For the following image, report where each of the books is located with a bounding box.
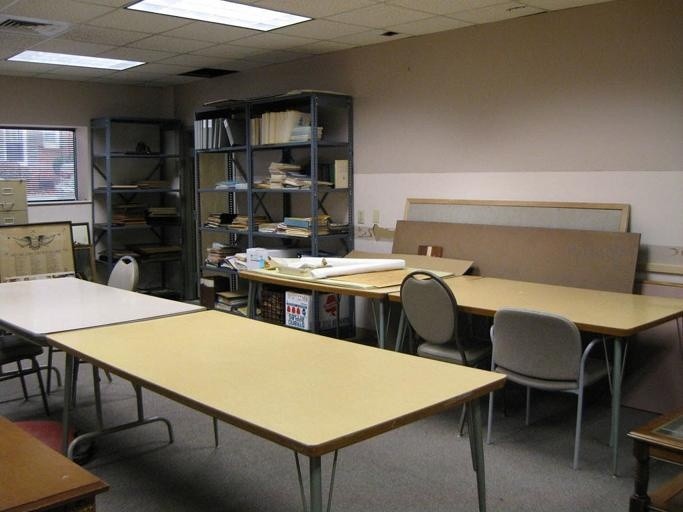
[191,111,346,319]
[97,179,185,302]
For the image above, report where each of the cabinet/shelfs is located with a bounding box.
[88,116,187,301]
[237,259,453,349]
[190,105,249,319]
[0,178,29,225]
[247,92,353,338]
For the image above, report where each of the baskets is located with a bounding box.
[261,288,284,323]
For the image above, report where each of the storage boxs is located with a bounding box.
[286,289,354,329]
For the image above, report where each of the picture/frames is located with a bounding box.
[402,196,633,235]
[0,221,78,279]
[74,245,97,284]
[71,221,91,247]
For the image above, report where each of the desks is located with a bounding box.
[389,271,681,478]
[624,403,682,511]
[47,308,507,507]
[0,412,113,510]
[0,274,207,449]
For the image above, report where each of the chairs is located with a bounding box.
[105,256,142,291]
[398,273,491,438]
[487,309,628,469]
[1,330,54,416]
